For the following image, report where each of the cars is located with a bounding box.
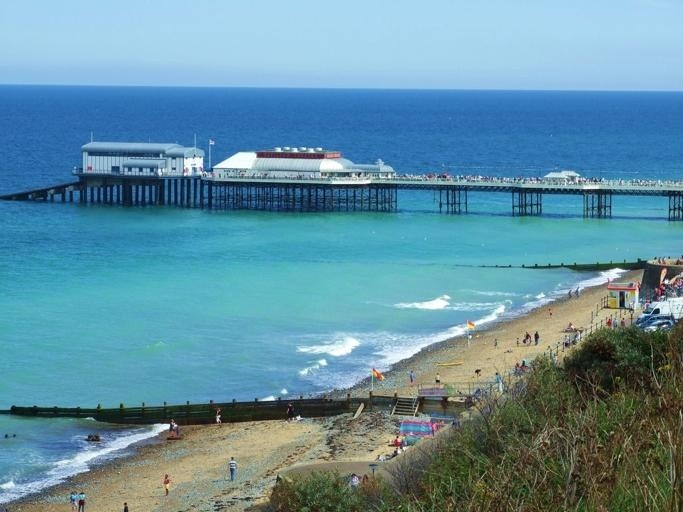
[635,286,682,334]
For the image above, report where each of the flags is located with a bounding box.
[372,367,384,381]
[467,320,475,330]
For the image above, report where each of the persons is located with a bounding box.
[376,435,406,461]
[216,408,222,424]
[169,419,181,438]
[496,372,503,394]
[123,503,128,512]
[70,491,86,512]
[431,422,437,437]
[409,370,414,386]
[472,369,481,378]
[435,373,441,384]
[494,331,539,376]
[163,473,170,496]
[228,457,239,481]
[549,256,683,349]
[286,402,294,422]
